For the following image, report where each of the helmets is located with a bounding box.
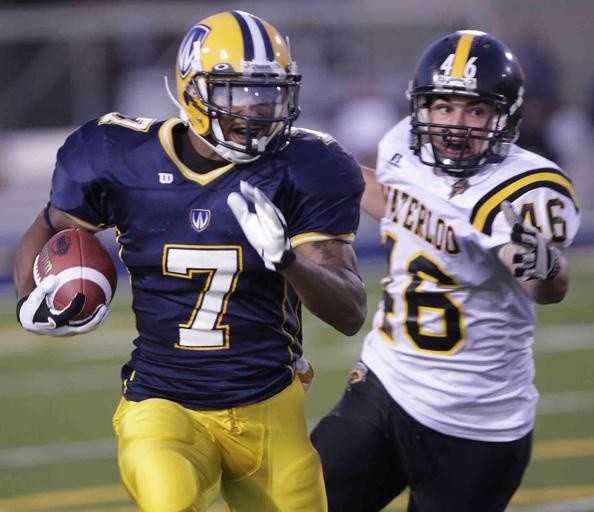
[407,28,527,172]
[176,10,301,163]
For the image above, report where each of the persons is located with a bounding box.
[11,9,367,512]
[307,30,583,512]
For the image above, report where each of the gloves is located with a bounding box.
[500,199,561,284]
[14,275,108,339]
[228,178,297,272]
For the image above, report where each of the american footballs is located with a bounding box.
[32,227,117,321]
